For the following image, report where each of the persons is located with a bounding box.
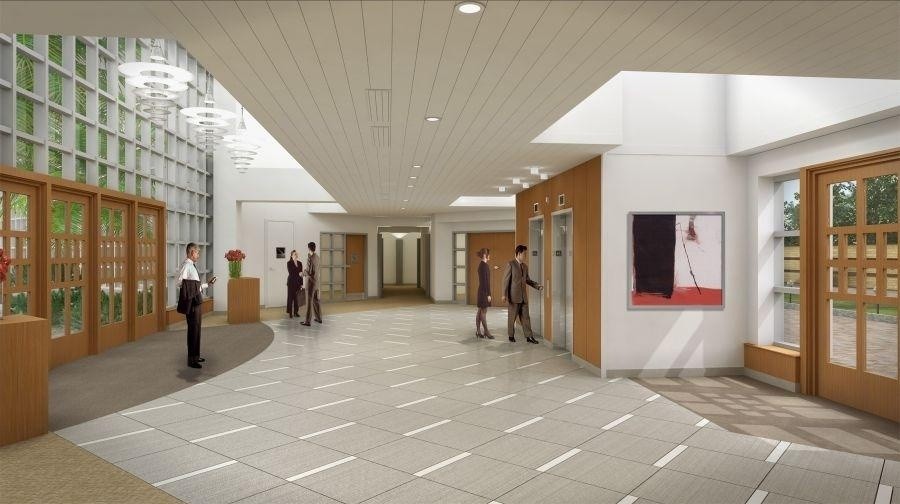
[178,242,209,370]
[287,250,303,319]
[501,245,543,344]
[475,248,494,339]
[300,242,323,327]
